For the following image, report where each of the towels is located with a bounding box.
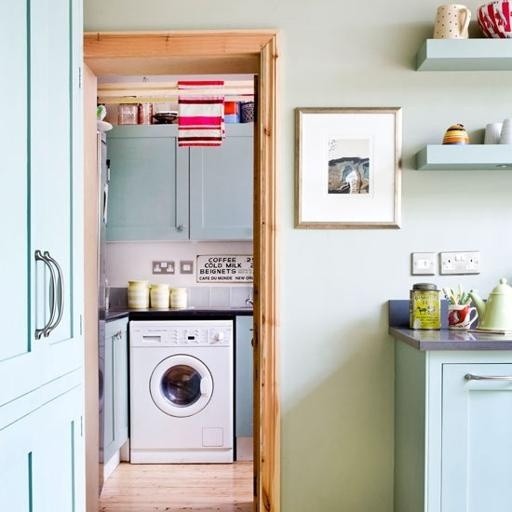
[177,80,225,147]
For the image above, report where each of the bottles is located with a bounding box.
[150,283,170,309]
[126,280,149,310]
[408,282,442,331]
[169,287,189,308]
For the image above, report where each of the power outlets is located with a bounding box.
[411,252,484,275]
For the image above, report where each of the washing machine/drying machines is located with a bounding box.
[129,319,235,465]
[98,320,106,463]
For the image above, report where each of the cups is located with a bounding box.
[482,123,501,144]
[446,304,481,332]
[498,117,512,144]
[431,3,472,40]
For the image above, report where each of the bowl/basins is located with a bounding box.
[441,122,469,145]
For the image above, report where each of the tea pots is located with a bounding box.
[466,276,511,334]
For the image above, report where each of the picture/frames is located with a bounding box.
[294,105,403,233]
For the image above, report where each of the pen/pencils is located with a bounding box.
[441,283,474,305]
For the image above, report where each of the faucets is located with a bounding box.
[245,300,253,304]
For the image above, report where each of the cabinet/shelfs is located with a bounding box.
[107,122,254,242]
[394,340,512,512]
[2,0,86,512]
